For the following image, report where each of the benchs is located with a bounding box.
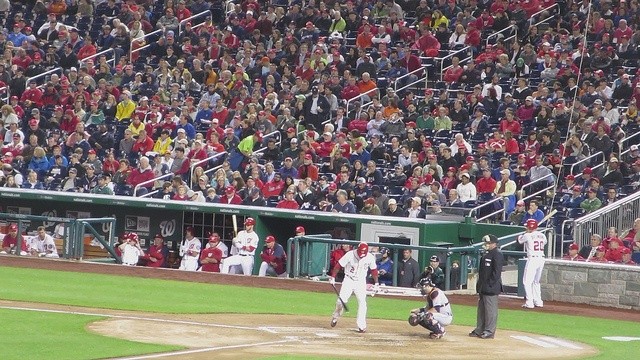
[0,236,117,264]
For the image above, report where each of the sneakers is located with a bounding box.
[522,304,534,309]
[481,333,494,339]
[430,331,446,339]
[536,305,543,308]
[469,331,479,337]
[359,327,366,333]
[331,318,337,327]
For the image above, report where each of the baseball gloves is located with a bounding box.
[408,312,427,326]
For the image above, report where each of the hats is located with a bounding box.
[542,42,550,46]
[411,197,421,204]
[525,96,533,101]
[620,248,630,254]
[307,124,316,131]
[90,102,97,106]
[264,236,274,242]
[305,154,312,159]
[462,173,470,178]
[287,127,296,134]
[11,96,18,100]
[406,121,416,127]
[211,38,218,44]
[13,134,20,138]
[249,158,258,163]
[212,119,218,124]
[4,151,13,158]
[330,183,337,189]
[177,139,188,145]
[481,168,491,174]
[129,233,138,241]
[482,234,499,242]
[408,129,415,134]
[573,186,580,191]
[447,167,456,172]
[389,199,396,205]
[596,246,605,251]
[580,169,591,174]
[68,27,81,32]
[324,132,332,137]
[122,234,128,239]
[68,168,77,173]
[296,226,304,232]
[424,90,432,94]
[505,93,513,98]
[29,120,38,127]
[594,99,603,106]
[568,244,577,249]
[630,145,638,151]
[564,175,574,179]
[420,278,436,286]
[355,142,361,149]
[225,187,234,192]
[428,155,437,160]
[358,243,368,257]
[89,150,96,154]
[11,225,17,231]
[594,69,604,76]
[524,219,537,229]
[247,3,256,11]
[149,113,157,117]
[258,111,266,116]
[466,156,475,162]
[154,233,162,238]
[425,142,431,147]
[244,218,255,225]
[357,178,365,184]
[590,178,599,182]
[210,236,217,241]
[607,237,619,241]
[58,32,64,36]
[364,198,374,203]
[597,116,604,122]
[587,189,597,193]
[429,256,439,261]
[610,157,617,162]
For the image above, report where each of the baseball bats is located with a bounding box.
[331,284,349,312]
[537,209,558,226]
[232,214,237,238]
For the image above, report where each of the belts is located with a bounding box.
[239,254,251,256]
[127,264,136,266]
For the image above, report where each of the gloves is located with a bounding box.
[330,278,335,286]
[417,312,428,322]
[411,309,420,314]
[370,283,379,296]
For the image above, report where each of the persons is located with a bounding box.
[0,0,640,226]
[31,226,59,258]
[469,234,503,339]
[330,239,353,281]
[373,247,393,286]
[450,260,461,291]
[223,218,260,276]
[142,234,169,268]
[409,278,453,339]
[115,233,145,266]
[0,224,26,256]
[563,218,640,267]
[258,236,287,277]
[518,219,547,308]
[397,248,421,288]
[419,255,445,290]
[197,232,228,273]
[113,231,130,256]
[329,241,379,333]
[178,227,201,271]
[291,227,305,272]
[198,237,222,273]
